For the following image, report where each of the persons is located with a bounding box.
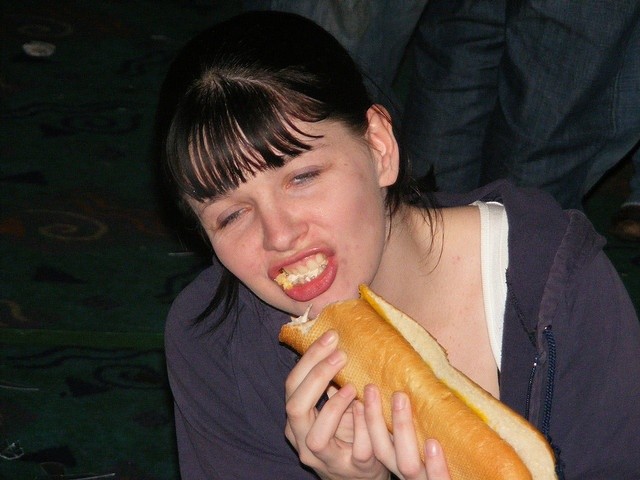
[164,11,640,479]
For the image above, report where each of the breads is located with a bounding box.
[278,282,559,480]
